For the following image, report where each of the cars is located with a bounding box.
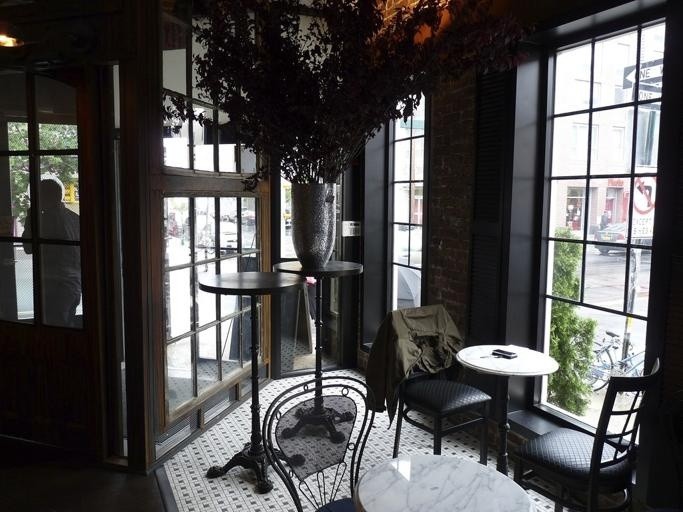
[595,224,627,253]
[242,212,255,226]
[168,213,237,255]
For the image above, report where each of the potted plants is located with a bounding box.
[167,0,455,269]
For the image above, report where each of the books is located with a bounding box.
[491,348,517,360]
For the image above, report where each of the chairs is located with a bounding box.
[522,358,661,512]
[367,303,493,464]
[262,376,377,512]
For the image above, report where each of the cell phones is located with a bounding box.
[492,348,517,359]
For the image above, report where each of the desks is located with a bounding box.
[354,454,530,512]
[199,260,363,494]
[455,344,560,475]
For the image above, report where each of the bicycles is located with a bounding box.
[574,330,645,391]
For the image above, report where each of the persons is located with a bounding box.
[599,208,609,231]
[570,200,581,222]
[20,175,82,329]
